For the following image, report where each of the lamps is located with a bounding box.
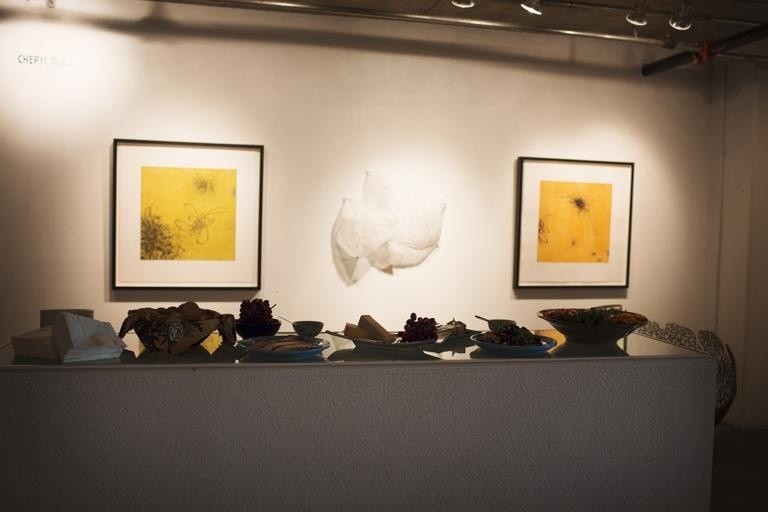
[624,1,693,31]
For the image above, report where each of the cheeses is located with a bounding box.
[437,321,467,341]
[345,315,396,344]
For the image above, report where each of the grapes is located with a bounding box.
[477,324,542,346]
[399,313,437,341]
[237,298,276,325]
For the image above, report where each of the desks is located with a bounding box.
[0,319,718,511]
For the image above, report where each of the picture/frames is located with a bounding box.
[516,157,634,288]
[112,138,261,287]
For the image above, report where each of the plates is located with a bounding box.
[330,313,437,354]
[473,315,555,354]
[239,333,329,360]
[437,320,484,345]
[537,307,648,346]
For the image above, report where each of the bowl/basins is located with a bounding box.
[234,319,281,339]
[291,318,323,338]
